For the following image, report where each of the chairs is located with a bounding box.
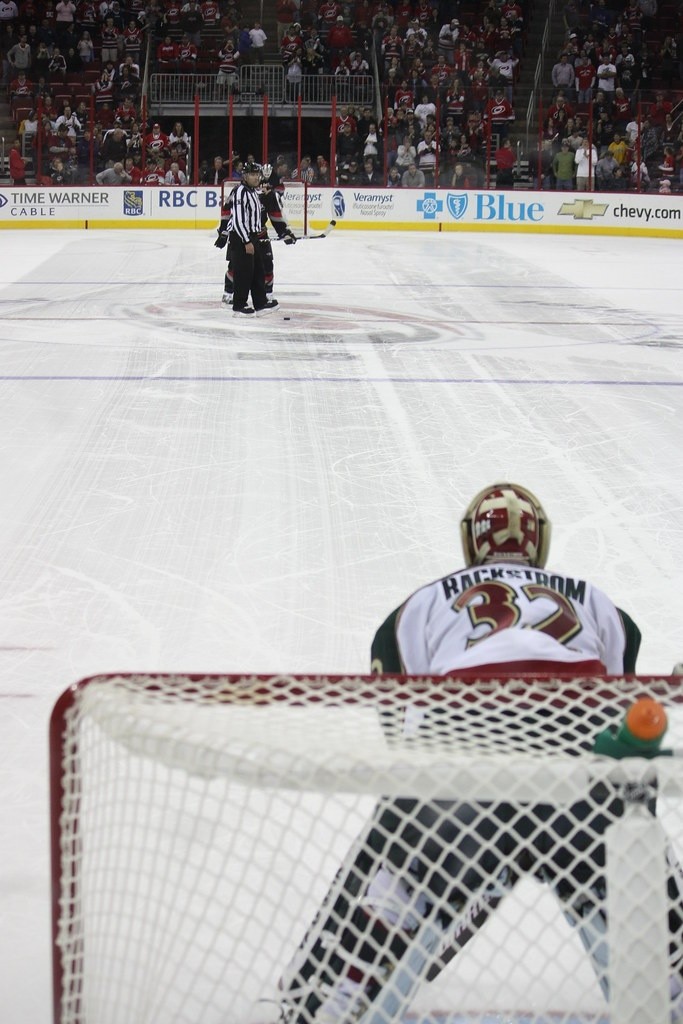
[0,0,683,191]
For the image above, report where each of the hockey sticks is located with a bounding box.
[259,219,336,243]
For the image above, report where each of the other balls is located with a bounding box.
[284,317,290,320]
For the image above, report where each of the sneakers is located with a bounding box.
[265,292,273,301]
[232,305,256,318]
[221,293,234,309]
[255,299,280,317]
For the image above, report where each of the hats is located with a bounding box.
[153,123,160,129]
[113,119,121,125]
[660,180,671,186]
[350,160,357,166]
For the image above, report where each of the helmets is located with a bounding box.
[459,483,552,569]
[240,162,262,174]
[261,164,272,178]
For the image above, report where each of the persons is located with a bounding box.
[278,483,683,1024]
[574,138,598,191]
[608,133,635,163]
[164,163,187,185]
[659,180,671,193]
[552,142,578,191]
[0,0,683,198]
[249,21,267,65]
[95,163,132,185]
[216,39,239,92]
[10,140,27,185]
[495,139,517,189]
[215,162,297,318]
[402,164,425,187]
[529,139,551,190]
[236,25,253,79]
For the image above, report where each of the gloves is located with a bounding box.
[214,231,228,248]
[277,191,284,197]
[282,230,296,244]
